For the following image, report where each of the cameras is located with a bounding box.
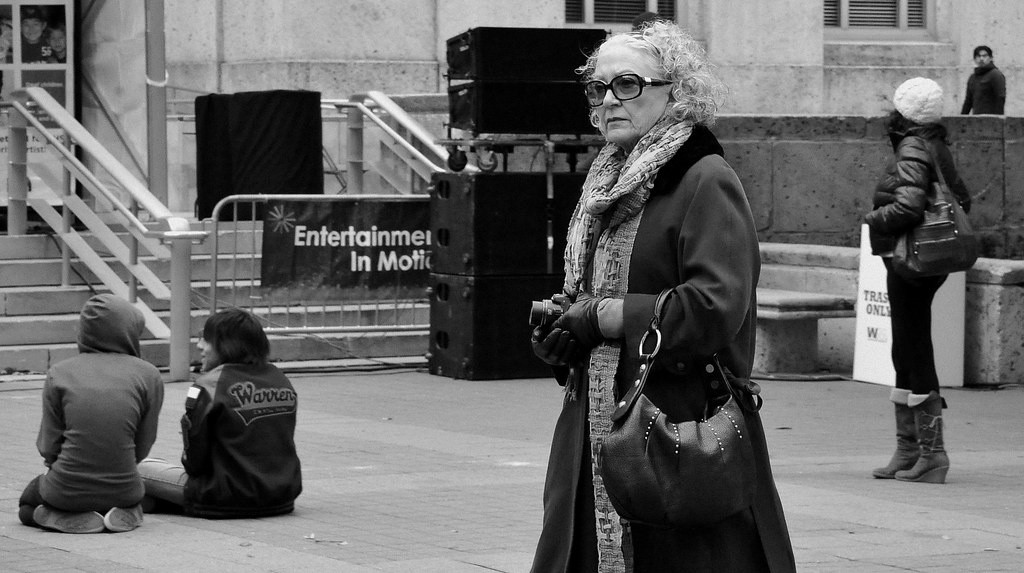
[530,296,573,339]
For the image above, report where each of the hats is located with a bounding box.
[892,76,946,126]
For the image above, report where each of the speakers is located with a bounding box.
[429,28,610,381]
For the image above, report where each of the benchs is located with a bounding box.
[756,288,856,374]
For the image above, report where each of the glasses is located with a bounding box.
[584,73,674,108]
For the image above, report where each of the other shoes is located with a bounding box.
[104,503,144,532]
[33,505,104,533]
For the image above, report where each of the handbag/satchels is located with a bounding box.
[597,286,763,530]
[892,135,979,281]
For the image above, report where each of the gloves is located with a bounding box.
[550,293,614,353]
[530,326,576,367]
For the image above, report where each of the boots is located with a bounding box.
[872,386,922,478]
[894,390,951,484]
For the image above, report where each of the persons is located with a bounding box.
[529,33,799,573]
[865,79,971,483]
[961,44,1007,116]
[135,308,303,520]
[18,294,164,532]
[20,6,68,65]
